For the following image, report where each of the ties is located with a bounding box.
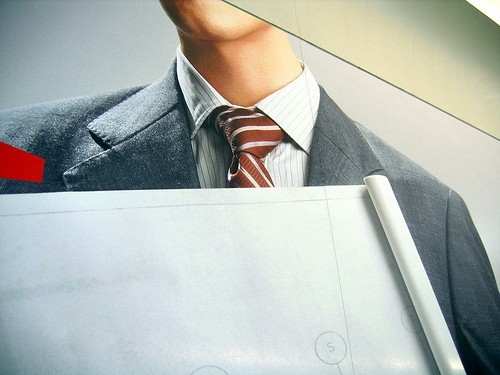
[201,104,288,187]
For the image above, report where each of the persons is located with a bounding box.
[0,0,500,374]
